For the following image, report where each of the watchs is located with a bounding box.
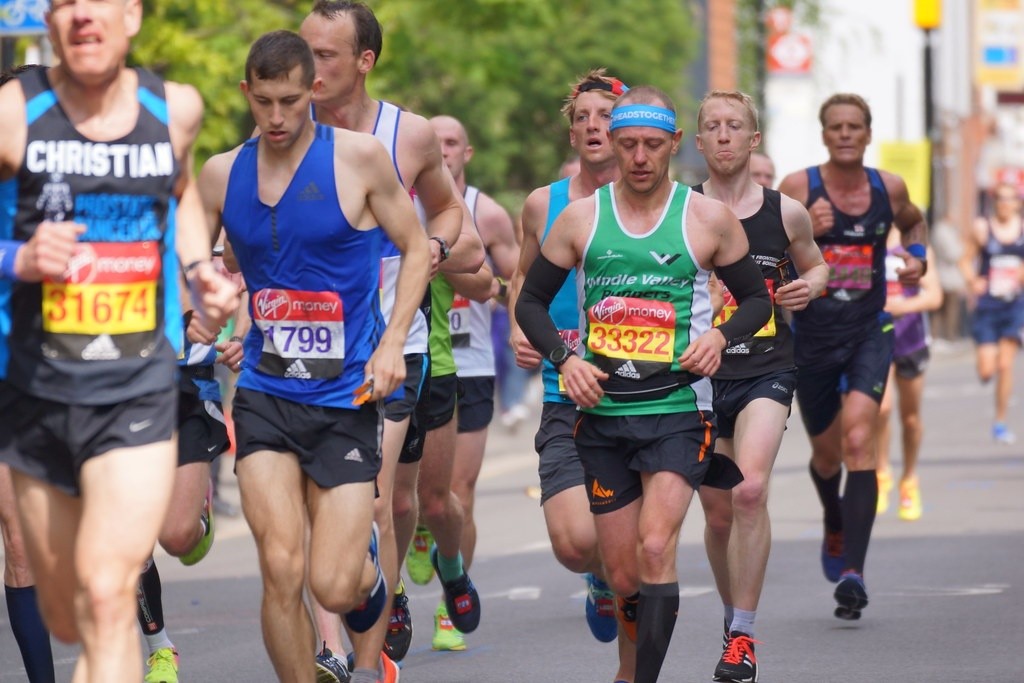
[549,344,578,374]
[429,237,450,263]
[494,277,507,302]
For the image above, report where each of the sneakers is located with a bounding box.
[383,576,413,662]
[430,543,480,634]
[179,477,216,566]
[432,601,466,651]
[833,570,869,620]
[712,616,759,683]
[820,497,847,582]
[145,648,180,683]
[343,520,388,633]
[586,572,617,642]
[406,524,436,585]
[876,470,893,515]
[897,475,921,521]
[616,591,641,643]
[315,640,403,683]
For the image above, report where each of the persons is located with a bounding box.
[509,67,942,683]
[0,0,520,683]
[958,183,1024,444]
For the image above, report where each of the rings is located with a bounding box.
[237,361,241,366]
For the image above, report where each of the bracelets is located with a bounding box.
[183,259,204,288]
[905,244,926,262]
[229,335,242,343]
[916,257,926,276]
[0,240,27,284]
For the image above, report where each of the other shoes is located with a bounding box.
[993,422,1012,445]
